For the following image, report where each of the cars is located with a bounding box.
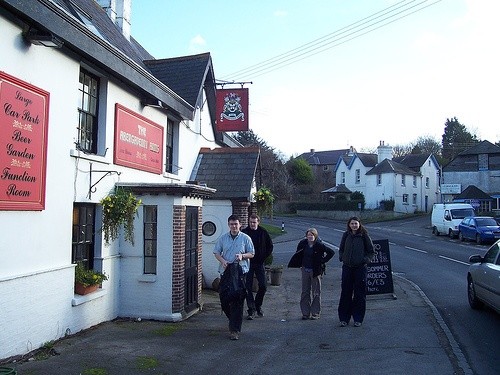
[459,216,500,245]
[466,239,500,314]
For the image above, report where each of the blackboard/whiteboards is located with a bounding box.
[366,239,394,296]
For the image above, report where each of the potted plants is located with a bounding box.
[269,264,284,286]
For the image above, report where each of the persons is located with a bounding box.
[241,214,273,320]
[214,215,255,339]
[338,217,374,328]
[288,228,335,320]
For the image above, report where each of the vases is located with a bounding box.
[75,282,99,296]
[259,200,271,207]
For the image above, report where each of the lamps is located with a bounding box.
[24,29,64,49]
[143,99,164,110]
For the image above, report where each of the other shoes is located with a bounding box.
[354,322,362,327]
[340,320,348,327]
[257,308,264,317]
[302,315,309,320]
[248,313,256,319]
[230,331,240,340]
[310,313,320,320]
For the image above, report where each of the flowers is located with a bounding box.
[74,264,110,287]
[95,192,141,247]
[253,186,278,204]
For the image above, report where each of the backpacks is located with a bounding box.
[219,259,248,301]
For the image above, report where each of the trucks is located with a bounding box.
[431,203,476,239]
